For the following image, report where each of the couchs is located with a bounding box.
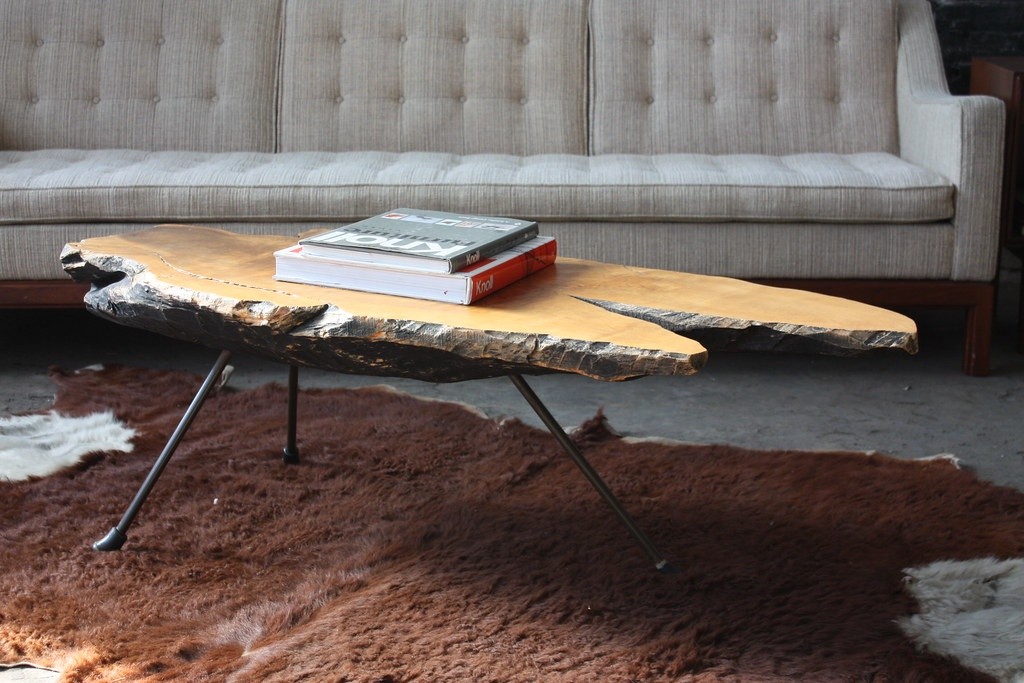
[0,0,1010,377]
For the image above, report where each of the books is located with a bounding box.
[275,205,559,306]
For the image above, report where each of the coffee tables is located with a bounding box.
[60,224,918,572]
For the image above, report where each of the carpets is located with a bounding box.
[0,359,1024,680]
